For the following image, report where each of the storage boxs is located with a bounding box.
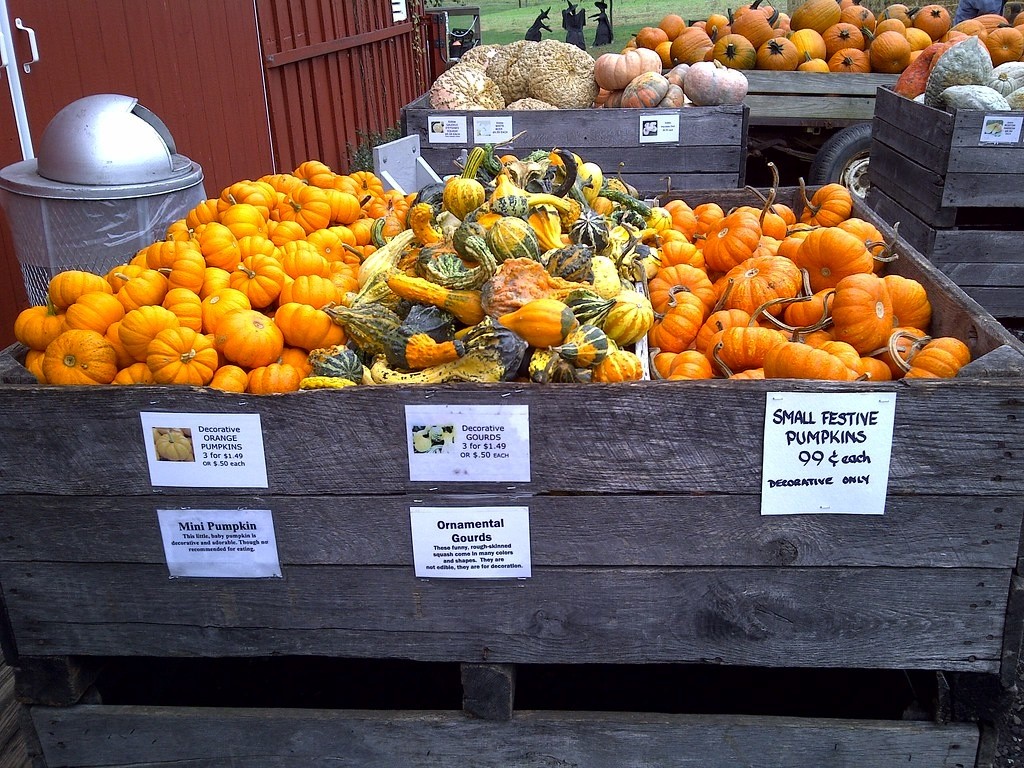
[2,81,1024,768]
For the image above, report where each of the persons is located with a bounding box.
[561,0,586,50]
[525,6,552,43]
[587,0,613,46]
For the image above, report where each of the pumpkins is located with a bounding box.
[429,0,1024,113]
[14,138,972,393]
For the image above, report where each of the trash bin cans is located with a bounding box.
[0,94,206,311]
